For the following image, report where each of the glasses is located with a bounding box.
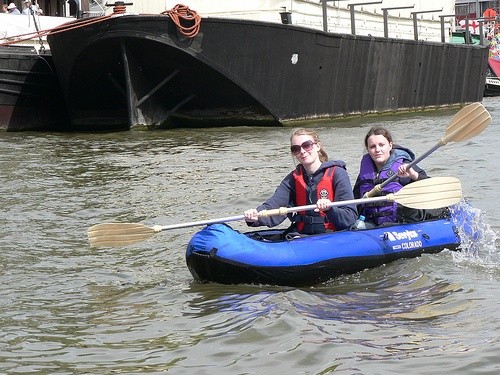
[291,140,317,155]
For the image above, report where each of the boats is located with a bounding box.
[46,0,491,129]
[185,202,482,290]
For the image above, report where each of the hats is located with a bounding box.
[24,0,31,3]
[8,3,17,9]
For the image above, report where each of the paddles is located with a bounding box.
[86,176,462,249]
[362,102,492,199]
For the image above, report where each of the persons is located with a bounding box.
[353,127,427,226]
[243,129,358,241]
[2,0,31,15]
